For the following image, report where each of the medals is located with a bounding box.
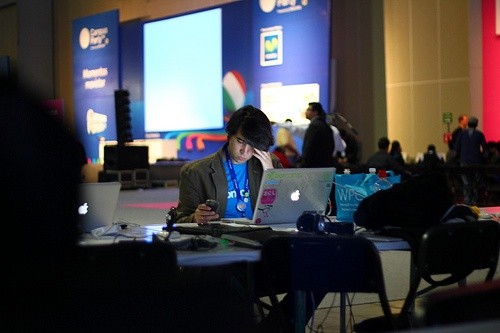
[236,201,247,212]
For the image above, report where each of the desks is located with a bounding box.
[80,216,410,333]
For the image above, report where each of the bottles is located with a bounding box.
[369,168,376,174]
[343,169,351,175]
[378,170,387,181]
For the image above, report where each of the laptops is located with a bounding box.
[221,166,337,225]
[78,181,127,234]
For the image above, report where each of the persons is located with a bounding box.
[175,105,292,224]
[366,137,412,174]
[415,115,500,205]
[301,101,362,173]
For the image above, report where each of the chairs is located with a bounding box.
[401,220,500,316]
[262,236,391,333]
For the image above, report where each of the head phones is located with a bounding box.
[296,213,354,235]
